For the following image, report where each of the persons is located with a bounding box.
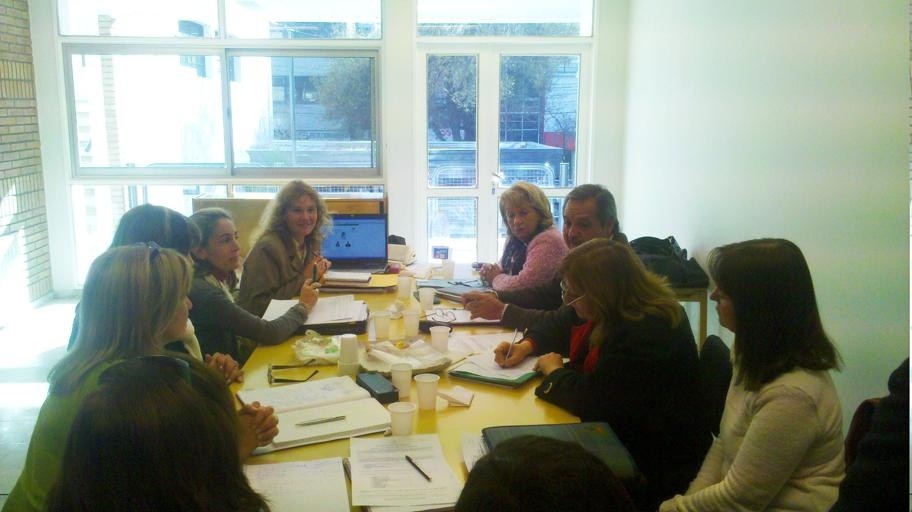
[659,238,850,510]
[43,241,280,512]
[462,183,631,328]
[185,210,321,365]
[453,432,638,512]
[497,235,708,497]
[46,355,268,511]
[237,178,331,331]
[469,182,568,293]
[107,205,245,391]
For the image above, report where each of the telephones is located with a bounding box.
[371,263,402,275]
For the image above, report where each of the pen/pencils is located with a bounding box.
[503,328,518,369]
[312,263,317,282]
[452,307,465,310]
[295,415,345,426]
[405,455,432,480]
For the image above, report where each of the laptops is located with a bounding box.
[318,213,388,274]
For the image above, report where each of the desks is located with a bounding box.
[672,286,708,358]
[228,276,582,512]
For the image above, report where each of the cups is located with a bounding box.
[429,326,451,350]
[374,312,390,339]
[403,309,419,337]
[398,276,411,296]
[390,363,412,398]
[387,402,417,436]
[443,261,454,280]
[338,334,360,373]
[420,288,434,308]
[414,373,440,412]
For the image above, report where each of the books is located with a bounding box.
[449,352,574,391]
[238,374,392,454]
[437,283,470,302]
[345,432,460,510]
[245,458,351,512]
[320,270,398,294]
[426,303,503,327]
[483,420,640,482]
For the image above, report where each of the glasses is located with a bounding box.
[421,308,457,323]
[134,240,163,262]
[561,280,588,307]
[266,359,320,385]
